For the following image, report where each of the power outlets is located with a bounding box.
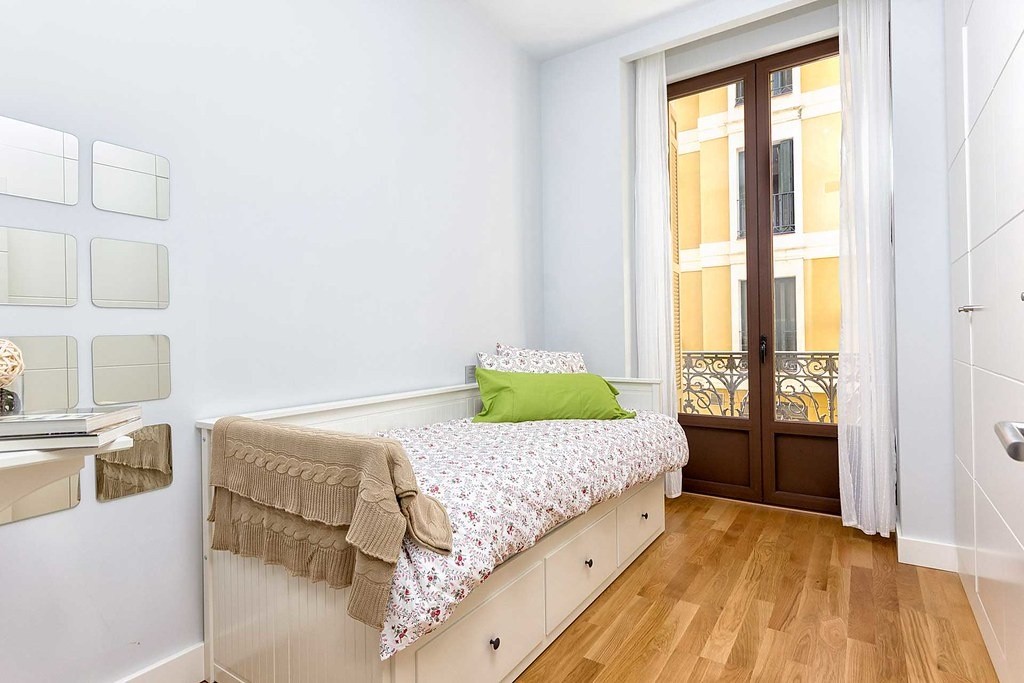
[466,365,476,383]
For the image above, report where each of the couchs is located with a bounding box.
[196,376,689,683]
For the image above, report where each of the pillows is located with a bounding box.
[472,343,636,422]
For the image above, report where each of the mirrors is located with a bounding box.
[91,334,170,405]
[0,336,79,413]
[0,115,79,205]
[0,472,82,526]
[90,237,170,308]
[91,139,170,220]
[1,226,78,307]
[96,424,174,502]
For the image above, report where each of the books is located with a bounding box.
[0,405,140,438]
[0,418,143,453]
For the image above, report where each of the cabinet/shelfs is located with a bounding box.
[946,0,1024,683]
